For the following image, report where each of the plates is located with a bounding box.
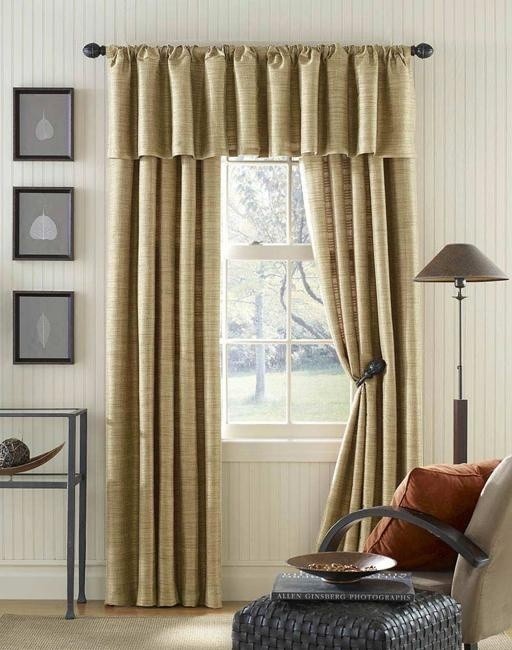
[286,551,398,583]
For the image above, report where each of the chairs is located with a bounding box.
[316,454,512,650]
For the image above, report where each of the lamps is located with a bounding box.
[412,243,509,466]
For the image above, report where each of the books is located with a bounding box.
[269,569,416,604]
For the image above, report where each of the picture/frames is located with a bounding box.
[12,87,74,364]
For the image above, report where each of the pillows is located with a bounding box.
[359,457,504,572]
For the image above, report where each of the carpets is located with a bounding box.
[0,609,241,649]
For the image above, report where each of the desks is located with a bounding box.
[231,582,463,649]
